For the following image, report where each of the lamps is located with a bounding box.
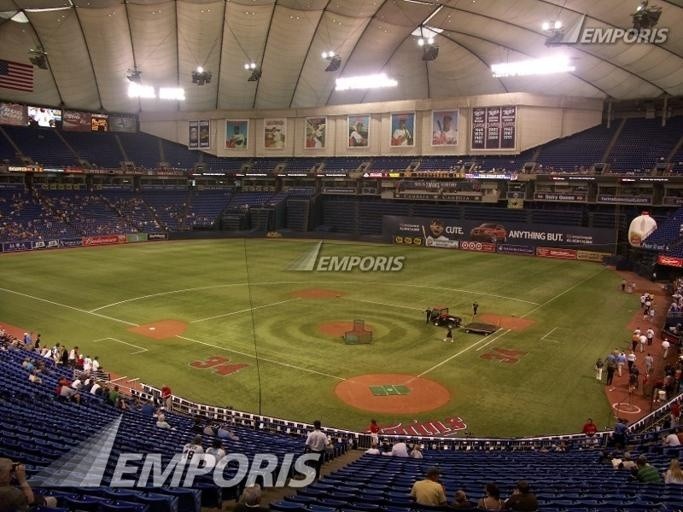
[543,0,662,49]
[127,66,263,86]
[28,49,49,71]
[421,47,439,61]
[324,55,341,72]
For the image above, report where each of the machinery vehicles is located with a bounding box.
[430,305,463,329]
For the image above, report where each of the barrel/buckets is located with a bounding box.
[627,211,657,248]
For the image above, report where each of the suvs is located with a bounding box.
[470,222,507,243]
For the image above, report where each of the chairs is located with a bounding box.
[0,114,683,258]
[0,345,683,512]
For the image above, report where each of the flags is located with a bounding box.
[0,59,33,93]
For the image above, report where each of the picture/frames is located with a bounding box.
[389,108,460,148]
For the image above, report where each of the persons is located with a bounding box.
[423,305,432,327]
[433,115,456,144]
[231,484,269,512]
[0,327,143,413]
[410,467,448,507]
[504,480,537,510]
[613,417,626,433]
[370,419,379,448]
[450,490,469,508]
[142,398,154,415]
[229,125,245,148]
[391,118,411,144]
[582,418,597,449]
[595,276,681,402]
[336,432,423,459]
[442,322,453,343]
[308,121,323,147]
[0,184,212,241]
[421,219,448,243]
[159,382,172,411]
[0,457,57,512]
[181,418,240,471]
[152,408,161,418]
[350,122,366,146]
[670,401,680,429]
[33,107,54,127]
[475,484,503,510]
[156,415,170,429]
[269,126,281,144]
[596,443,682,485]
[304,420,335,478]
[660,429,680,448]
[470,300,477,319]
